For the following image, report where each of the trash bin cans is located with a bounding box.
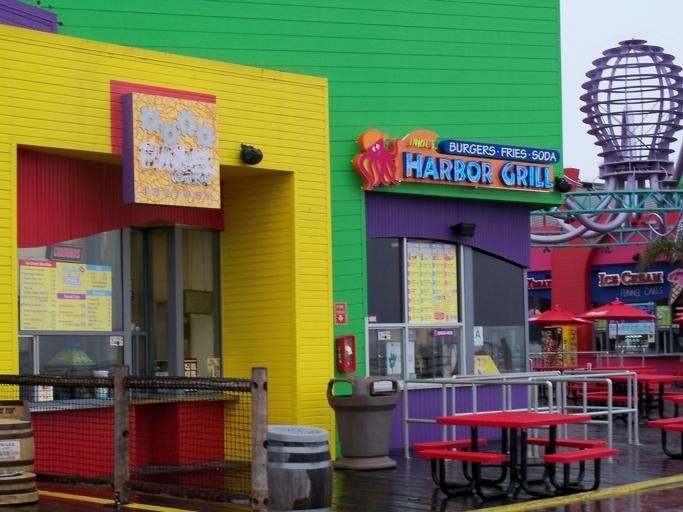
[327,375,400,470]
[263,424,333,512]
[0,400,39,512]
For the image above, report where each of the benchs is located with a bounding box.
[412,369,683,503]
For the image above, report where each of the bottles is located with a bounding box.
[545,328,559,366]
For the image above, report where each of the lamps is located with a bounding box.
[450,223,476,237]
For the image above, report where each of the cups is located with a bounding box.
[586,362,591,370]
[93,370,109,400]
